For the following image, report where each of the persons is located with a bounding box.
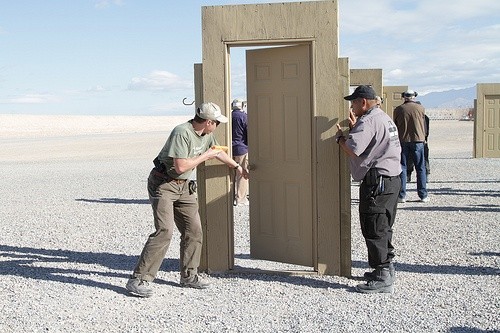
[229,99,249,205]
[125,103,249,296]
[391,89,431,203]
[337,85,404,293]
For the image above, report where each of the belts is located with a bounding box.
[155,170,184,184]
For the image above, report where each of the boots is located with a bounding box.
[355,263,396,294]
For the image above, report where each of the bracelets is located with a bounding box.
[233,164,240,170]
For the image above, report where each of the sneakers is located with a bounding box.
[178,274,210,289]
[126,275,154,297]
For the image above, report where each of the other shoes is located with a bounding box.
[398,196,406,204]
[420,197,430,202]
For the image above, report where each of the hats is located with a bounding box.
[233,99,242,109]
[196,102,229,124]
[343,85,377,101]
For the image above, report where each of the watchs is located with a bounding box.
[336,136,342,144]
